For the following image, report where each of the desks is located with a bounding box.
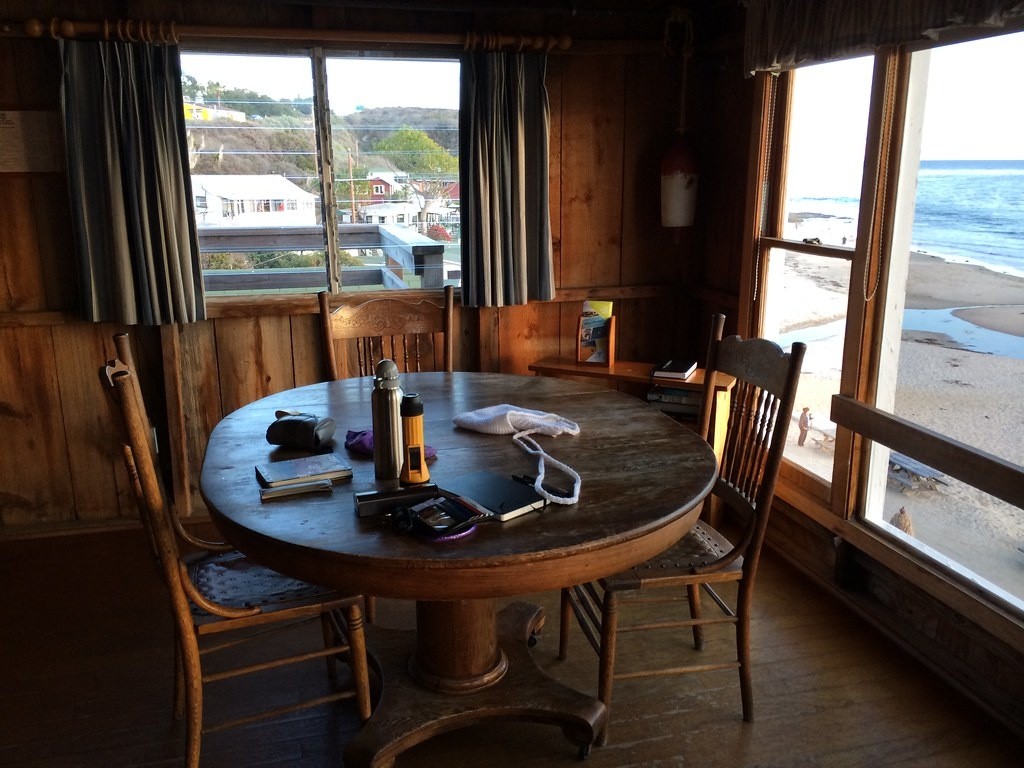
[818,428,836,450]
[889,452,945,495]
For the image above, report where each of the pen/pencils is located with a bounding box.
[511,473,535,487]
[522,473,571,496]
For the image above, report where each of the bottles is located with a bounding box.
[371,359,404,479]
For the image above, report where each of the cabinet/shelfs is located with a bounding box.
[527,355,738,534]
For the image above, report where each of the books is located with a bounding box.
[643,384,706,424]
[254,451,355,493]
[650,357,699,380]
[435,470,556,523]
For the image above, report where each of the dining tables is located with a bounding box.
[199,370,719,768]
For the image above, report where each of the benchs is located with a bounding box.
[811,436,836,452]
[888,466,950,490]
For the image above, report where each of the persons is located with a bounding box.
[797,407,813,446]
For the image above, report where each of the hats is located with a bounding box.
[455,404,579,507]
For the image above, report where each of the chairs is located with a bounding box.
[313,284,454,382]
[560,313,809,751]
[97,330,371,767]
